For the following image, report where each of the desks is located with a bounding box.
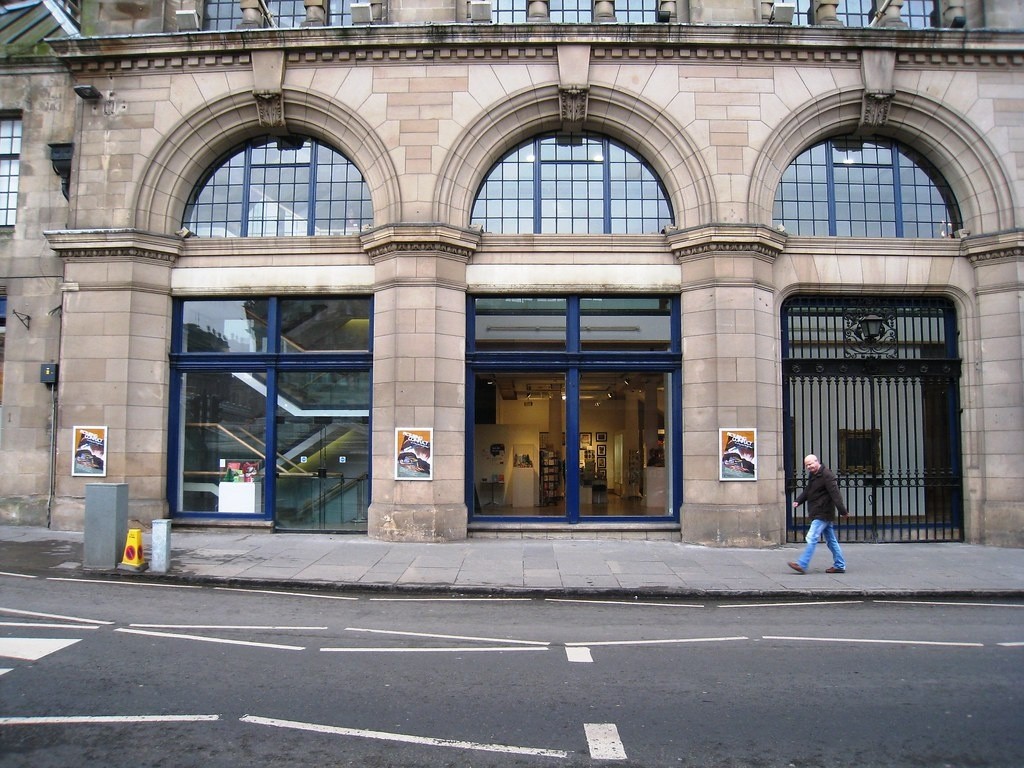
[512,467,534,507]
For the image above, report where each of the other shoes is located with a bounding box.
[826,566,845,572]
[787,562,805,573]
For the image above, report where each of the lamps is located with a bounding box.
[624,378,630,385]
[656,11,671,23]
[769,3,796,25]
[73,85,104,100]
[950,16,967,29]
[349,3,375,27]
[607,392,615,397]
[471,1,492,24]
[176,10,201,33]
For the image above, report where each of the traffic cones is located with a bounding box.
[117,528,148,570]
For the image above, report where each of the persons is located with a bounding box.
[647,436,665,467]
[788,454,850,575]
[242,461,251,474]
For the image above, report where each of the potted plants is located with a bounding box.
[647,448,665,467]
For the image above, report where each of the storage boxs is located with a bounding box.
[544,467,558,473]
[544,451,554,457]
[544,491,558,497]
[544,482,559,490]
[544,459,558,465]
[543,475,558,481]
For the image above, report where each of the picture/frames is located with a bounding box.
[72,427,107,476]
[580,432,592,446]
[599,469,607,476]
[394,428,433,481]
[597,457,606,468]
[597,445,606,456]
[596,432,607,442]
[718,428,758,482]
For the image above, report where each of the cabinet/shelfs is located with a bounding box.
[540,451,560,500]
